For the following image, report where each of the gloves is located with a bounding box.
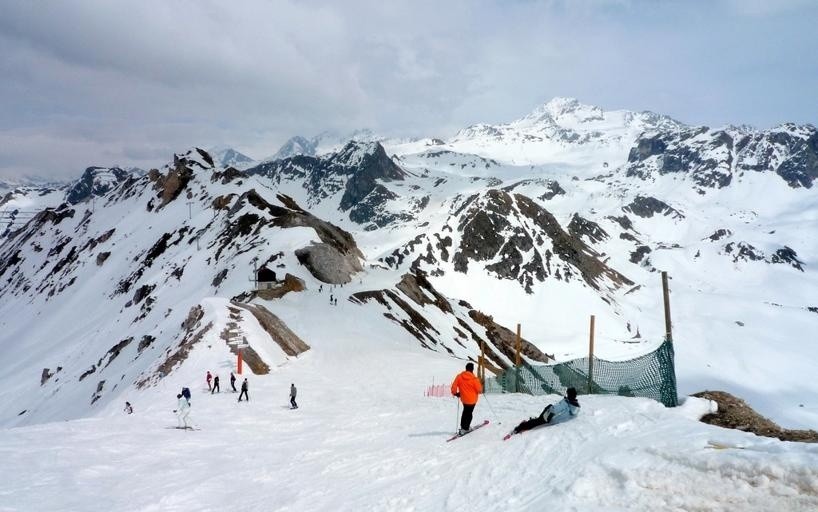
[455,392,460,397]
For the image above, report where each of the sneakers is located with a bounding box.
[462,427,472,433]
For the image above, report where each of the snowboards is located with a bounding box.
[504,419,527,440]
[447,420,489,442]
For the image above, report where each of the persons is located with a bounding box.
[451,364,484,436]
[239,378,248,401]
[124,402,132,414]
[212,376,219,394]
[290,383,298,408]
[513,387,581,434]
[206,370,212,390]
[231,372,236,391]
[173,394,195,430]
